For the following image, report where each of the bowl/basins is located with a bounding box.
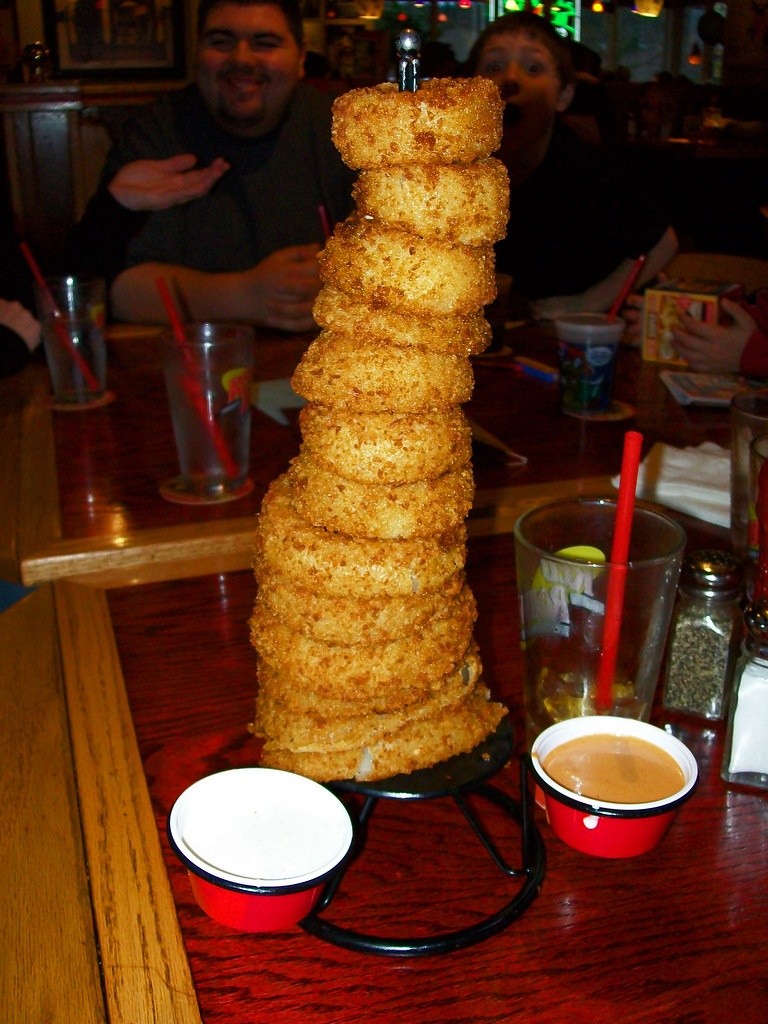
[168,766,355,934]
[530,715,698,860]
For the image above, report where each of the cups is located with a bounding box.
[729,392,768,610]
[553,312,625,414]
[749,435,768,598]
[156,325,256,496]
[483,274,513,352]
[34,275,108,403]
[514,495,688,752]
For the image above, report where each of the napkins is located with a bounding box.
[611,440,732,529]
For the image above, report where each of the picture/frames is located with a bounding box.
[41,0,189,83]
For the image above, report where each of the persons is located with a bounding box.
[387,26,768,148]
[0,154,230,379]
[623,283,768,381]
[467,12,679,319]
[78,0,358,334]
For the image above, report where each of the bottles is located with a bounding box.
[721,600,768,789]
[660,550,743,724]
